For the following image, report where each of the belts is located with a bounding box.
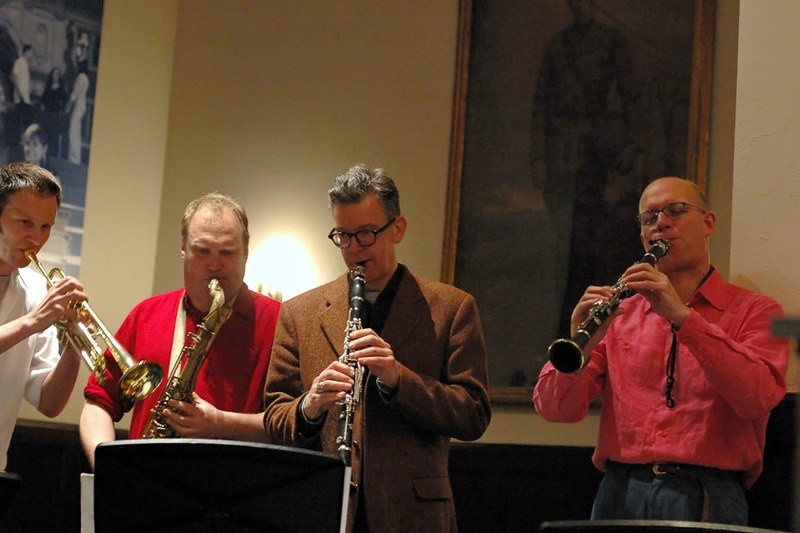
[613,462,735,521]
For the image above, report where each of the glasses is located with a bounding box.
[329,218,395,249]
[636,202,707,227]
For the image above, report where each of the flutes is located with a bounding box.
[548,236,669,375]
[334,262,367,497]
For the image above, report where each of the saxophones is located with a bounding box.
[139,277,225,438]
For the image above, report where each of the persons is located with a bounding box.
[0,163,97,473]
[79,194,282,473]
[532,177,791,526]
[263,163,492,533]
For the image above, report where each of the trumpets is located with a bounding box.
[26,248,165,401]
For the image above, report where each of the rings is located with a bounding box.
[317,382,324,393]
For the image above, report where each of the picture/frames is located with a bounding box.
[439,0,716,404]
